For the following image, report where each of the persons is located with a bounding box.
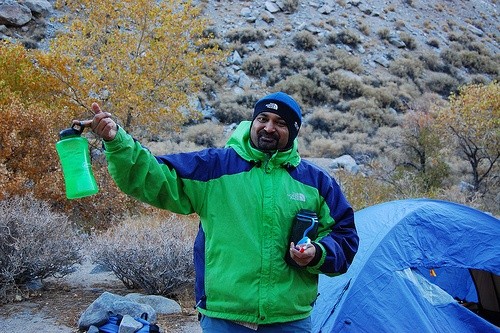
[71,92,359,332]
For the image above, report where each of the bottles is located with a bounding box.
[56,122,100,201]
[283,209,321,269]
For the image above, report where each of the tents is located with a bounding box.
[308,198,500,332]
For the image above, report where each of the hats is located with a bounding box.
[250,92,302,147]
